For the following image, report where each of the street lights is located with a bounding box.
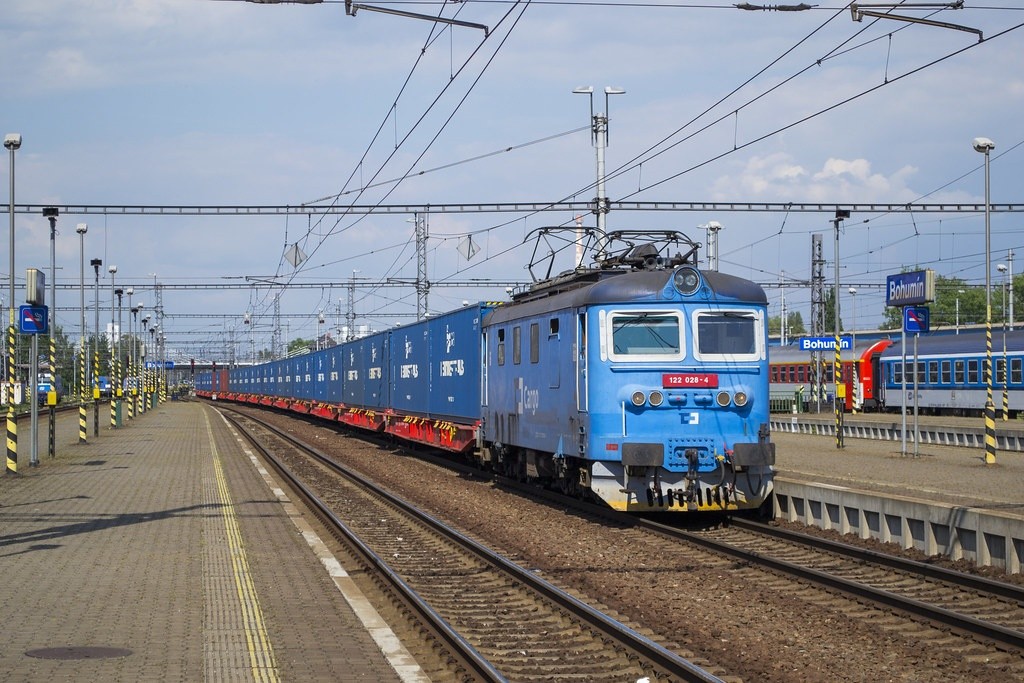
[846,286,858,415]
[108,264,118,428]
[970,135,996,464]
[41,206,61,460]
[3,132,23,475]
[572,83,626,261]
[995,263,1012,421]
[89,259,103,436]
[128,287,169,421]
[115,290,126,427]
[76,223,88,444]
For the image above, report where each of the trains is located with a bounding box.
[191,221,776,517]
[768,329,1024,421]
